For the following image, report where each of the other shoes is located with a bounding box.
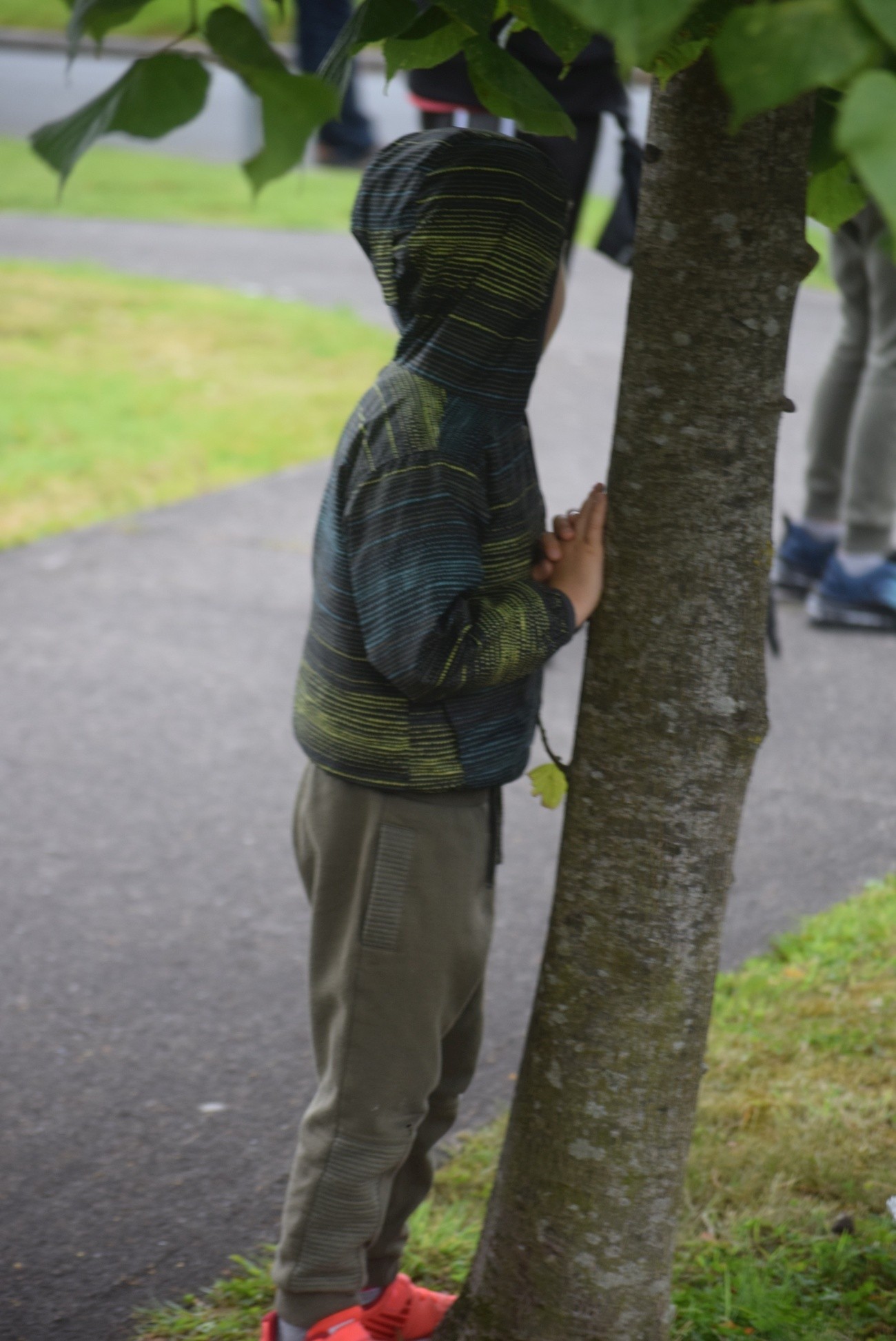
[316,137,381,168]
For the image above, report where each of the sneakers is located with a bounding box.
[260,1304,373,1341]
[803,548,896,628]
[363,1271,458,1341]
[776,516,839,589]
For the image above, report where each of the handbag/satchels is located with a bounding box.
[597,137,643,266]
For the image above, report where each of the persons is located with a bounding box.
[772,202,895,632]
[257,125,607,1341]
